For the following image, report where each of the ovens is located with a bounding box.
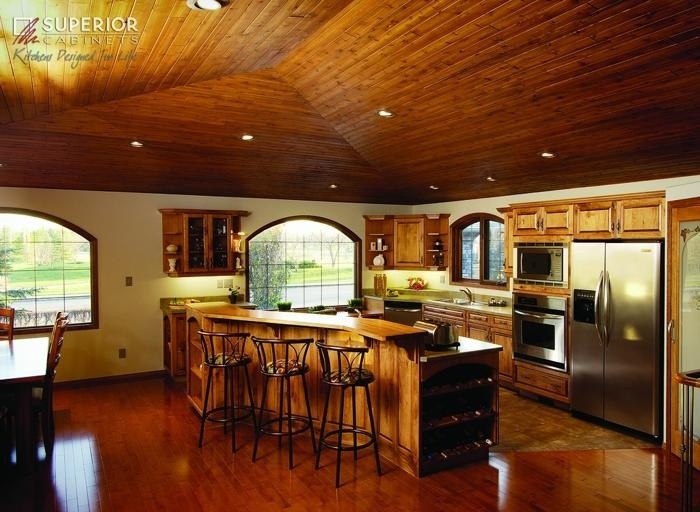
[511,294,568,373]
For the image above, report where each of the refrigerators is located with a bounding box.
[567,242,664,445]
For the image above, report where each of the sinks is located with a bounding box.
[459,299,484,308]
[433,298,466,303]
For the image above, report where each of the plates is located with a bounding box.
[373,256,385,266]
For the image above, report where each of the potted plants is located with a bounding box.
[228,286,241,303]
[308,305,336,315]
[277,301,292,312]
[345,298,367,313]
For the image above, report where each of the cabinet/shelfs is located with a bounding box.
[391,351,500,478]
[186,306,239,422]
[159,208,250,278]
[362,214,451,271]
[467,313,513,389]
[422,305,466,342]
[163,313,185,377]
[496,191,666,277]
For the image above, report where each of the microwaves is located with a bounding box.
[517,245,568,288]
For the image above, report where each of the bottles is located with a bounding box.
[222,218,227,234]
[373,273,387,297]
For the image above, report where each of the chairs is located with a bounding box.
[252,336,317,469]
[316,340,380,488]
[0,308,69,465]
[198,329,258,452]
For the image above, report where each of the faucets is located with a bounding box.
[459,288,472,301]
